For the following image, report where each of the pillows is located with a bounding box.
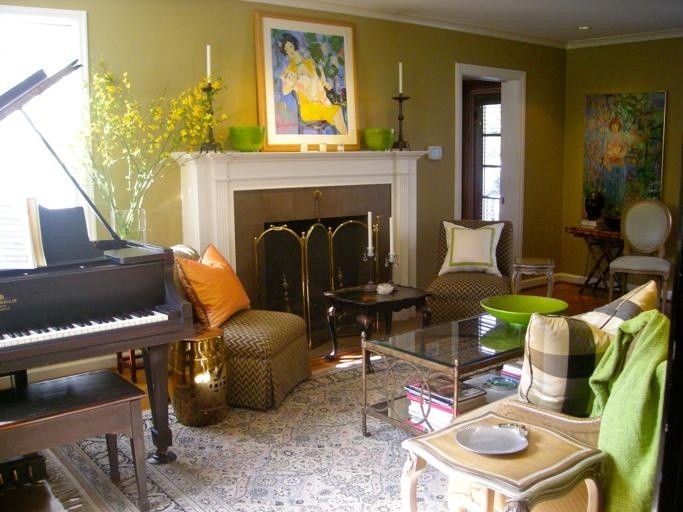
[174,242,252,328]
[438,220,506,280]
[517,278,658,419]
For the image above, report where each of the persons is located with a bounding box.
[279,33,346,134]
[601,118,630,211]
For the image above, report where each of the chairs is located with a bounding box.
[418,219,513,371]
[170,244,311,414]
[606,199,672,313]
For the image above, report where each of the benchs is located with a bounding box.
[0,365,150,510]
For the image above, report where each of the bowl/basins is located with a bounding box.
[230,126,265,152]
[364,128,395,151]
[478,324,538,352]
[477,294,569,326]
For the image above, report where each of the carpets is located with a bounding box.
[40,334,517,512]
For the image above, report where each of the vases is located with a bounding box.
[111,208,147,247]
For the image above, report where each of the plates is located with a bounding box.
[454,423,528,456]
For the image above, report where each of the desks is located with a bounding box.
[397,411,607,512]
[565,223,624,295]
[320,281,433,375]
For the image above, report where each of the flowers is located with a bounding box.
[79,52,235,209]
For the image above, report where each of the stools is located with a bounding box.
[115,347,144,381]
[170,326,228,428]
[510,256,555,297]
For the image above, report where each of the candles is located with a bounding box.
[366,210,375,256]
[205,43,213,82]
[387,215,395,261]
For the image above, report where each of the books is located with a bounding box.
[499,361,522,378]
[0,194,46,272]
[405,373,488,433]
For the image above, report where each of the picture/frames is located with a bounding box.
[397,63,403,95]
[252,8,362,155]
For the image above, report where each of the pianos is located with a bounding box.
[0,59,195,465]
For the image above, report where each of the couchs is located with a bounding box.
[447,306,669,512]
